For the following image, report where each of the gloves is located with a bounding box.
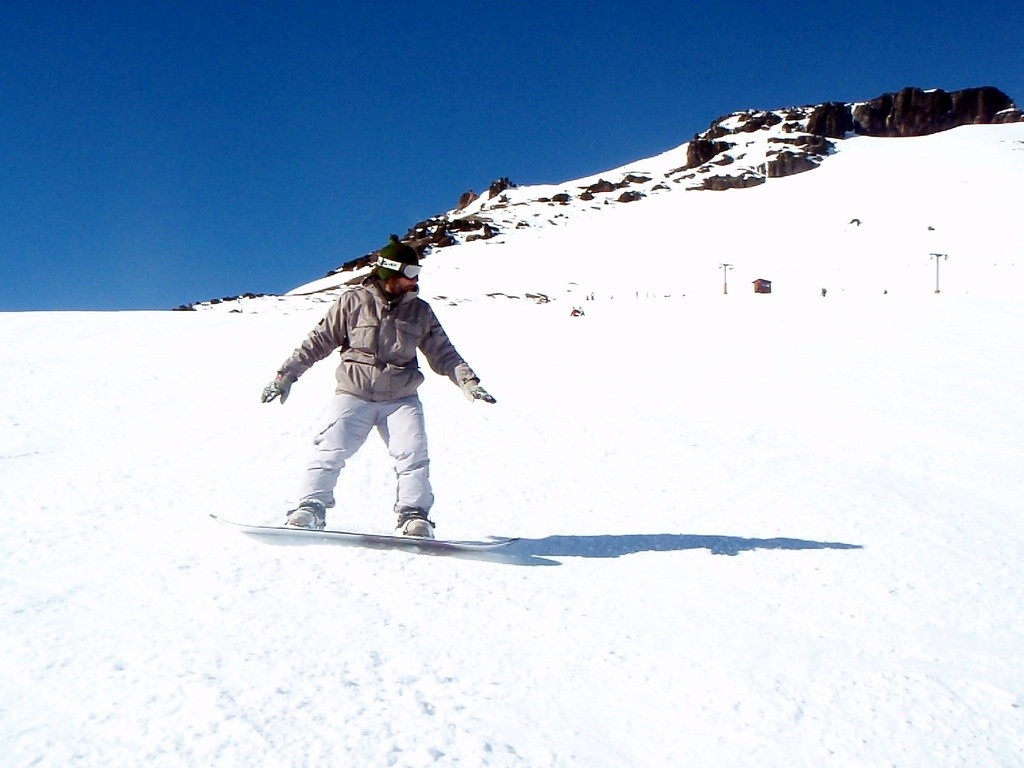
[464,380,496,404]
[262,366,299,405]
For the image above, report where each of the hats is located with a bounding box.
[374,234,419,283]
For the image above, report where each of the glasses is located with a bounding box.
[376,255,422,280]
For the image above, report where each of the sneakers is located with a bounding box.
[396,506,436,536]
[285,499,326,531]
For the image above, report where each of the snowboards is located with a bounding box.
[208,510,522,553]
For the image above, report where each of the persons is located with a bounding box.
[262,234,497,536]
